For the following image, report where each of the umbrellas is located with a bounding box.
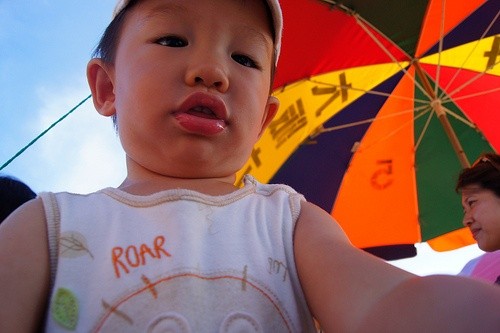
[235,1,500,262]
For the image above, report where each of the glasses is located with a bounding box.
[471,154,500,178]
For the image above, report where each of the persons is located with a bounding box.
[0,1,500,333]
[454,151,500,285]
